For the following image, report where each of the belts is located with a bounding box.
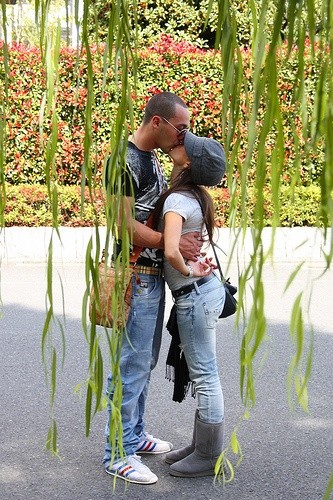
[110,259,164,278]
[171,270,217,298]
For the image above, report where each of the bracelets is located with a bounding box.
[182,265,194,278]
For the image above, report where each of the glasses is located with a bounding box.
[155,115,190,135]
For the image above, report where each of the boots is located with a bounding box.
[164,409,199,464]
[168,417,226,478]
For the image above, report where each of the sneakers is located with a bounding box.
[104,453,158,484]
[134,434,173,454]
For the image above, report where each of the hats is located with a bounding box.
[184,132,226,186]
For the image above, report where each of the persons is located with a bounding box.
[158,132,227,478]
[101,92,190,484]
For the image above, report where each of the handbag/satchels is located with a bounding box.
[218,277,237,319]
[88,263,140,328]
